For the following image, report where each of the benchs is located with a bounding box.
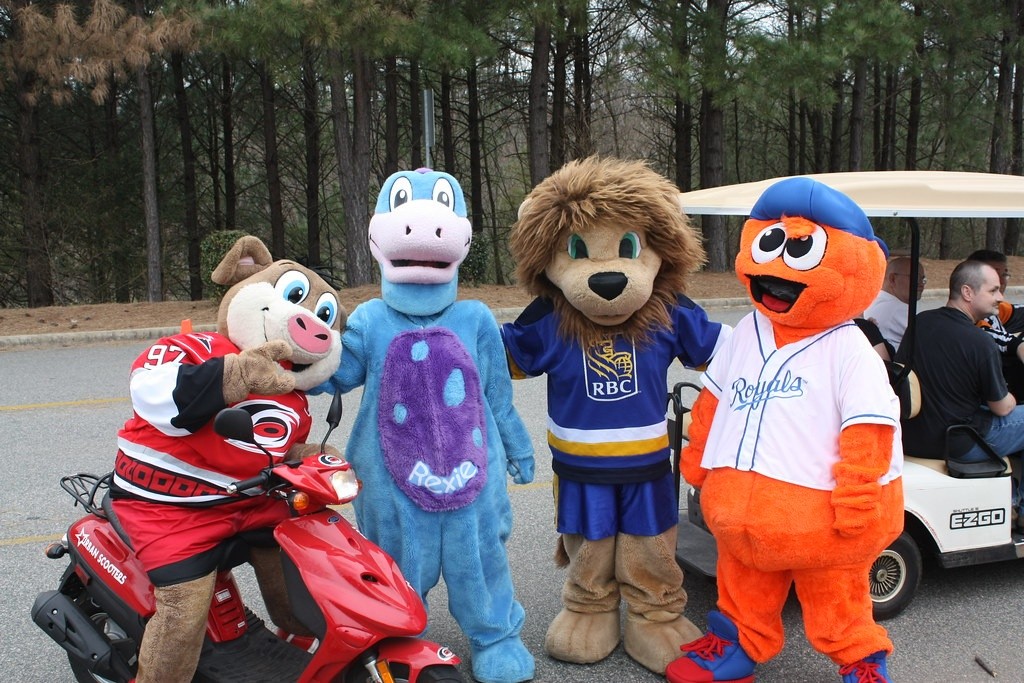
[883,359,1013,476]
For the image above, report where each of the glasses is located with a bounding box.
[1001,273,1013,283]
[895,273,928,285]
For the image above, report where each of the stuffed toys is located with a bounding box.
[109,234,344,683]
[498,152,734,676]
[305,168,536,683]
[666,178,904,683]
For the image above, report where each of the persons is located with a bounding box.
[863,250,1024,536]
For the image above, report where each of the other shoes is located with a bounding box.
[1017,516,1024,535]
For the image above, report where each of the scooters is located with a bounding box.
[31,390,466,683]
